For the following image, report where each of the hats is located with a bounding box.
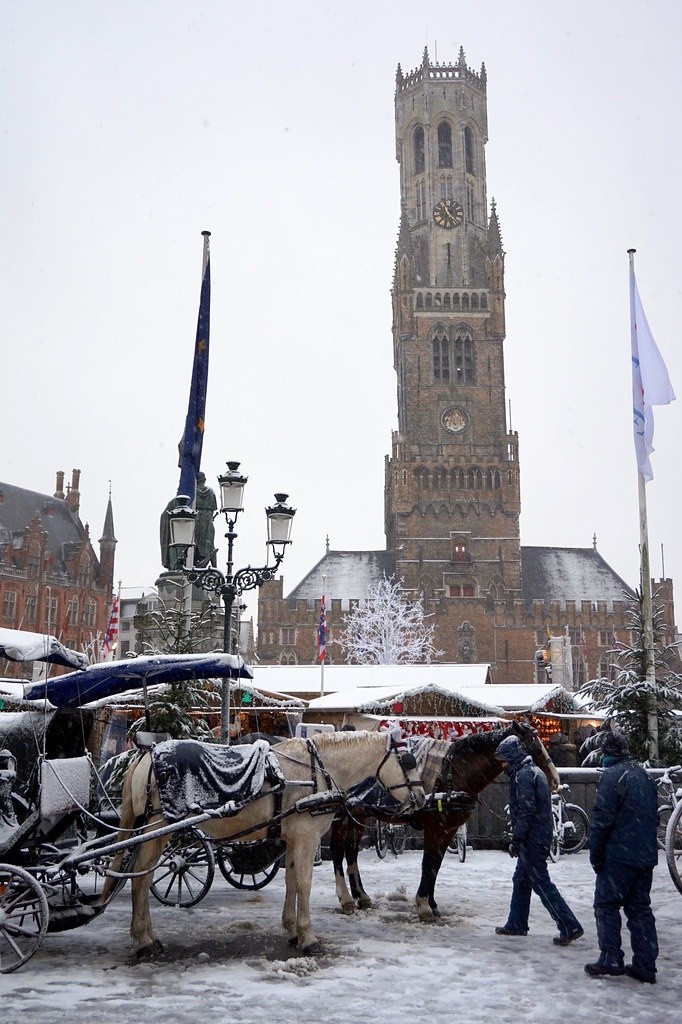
[601,732,628,757]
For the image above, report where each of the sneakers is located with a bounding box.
[585,961,625,976]
[496,922,528,936]
[553,927,585,946]
[626,963,657,984]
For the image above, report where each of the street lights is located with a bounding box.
[165,460,297,744]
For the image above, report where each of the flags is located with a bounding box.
[319,591,327,662]
[630,262,676,485]
[177,253,212,503]
[580,628,591,662]
[101,592,120,659]
[541,624,553,664]
[612,632,619,652]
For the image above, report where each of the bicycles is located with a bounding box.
[641,759,682,894]
[374,797,406,859]
[446,822,466,863]
[504,784,590,863]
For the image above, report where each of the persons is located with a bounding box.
[583,732,660,985]
[494,735,585,947]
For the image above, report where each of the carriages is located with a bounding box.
[0,588,560,974]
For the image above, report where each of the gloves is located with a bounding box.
[591,854,606,873]
[509,844,519,858]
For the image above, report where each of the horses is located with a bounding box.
[330,718,562,923]
[99,726,426,961]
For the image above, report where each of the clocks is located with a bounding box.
[433,197,464,228]
